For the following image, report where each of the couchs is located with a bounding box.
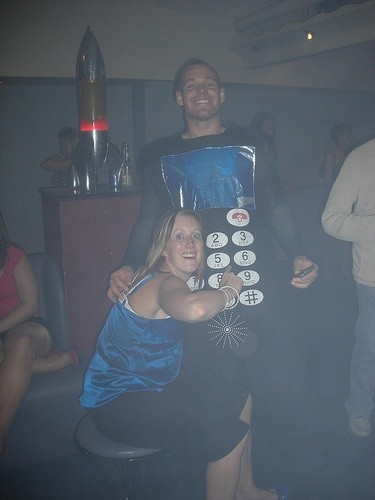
[0,252,94,471]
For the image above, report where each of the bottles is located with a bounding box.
[66,162,81,194]
[116,143,134,195]
[80,161,97,194]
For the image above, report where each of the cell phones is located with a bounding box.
[293,265,314,279]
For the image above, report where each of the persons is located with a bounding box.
[41,127,82,188]
[324,123,354,183]
[320,137,375,439]
[104,59,318,499]
[252,110,279,169]
[0,211,84,448]
[76,205,292,500]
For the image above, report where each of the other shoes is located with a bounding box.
[276,489,289,499]
[50,349,79,367]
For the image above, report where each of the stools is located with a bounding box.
[74,414,163,500]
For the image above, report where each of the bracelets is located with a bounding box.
[219,285,240,313]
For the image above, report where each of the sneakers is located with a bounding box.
[347,415,370,436]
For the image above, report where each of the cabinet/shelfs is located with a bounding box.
[38,186,143,366]
